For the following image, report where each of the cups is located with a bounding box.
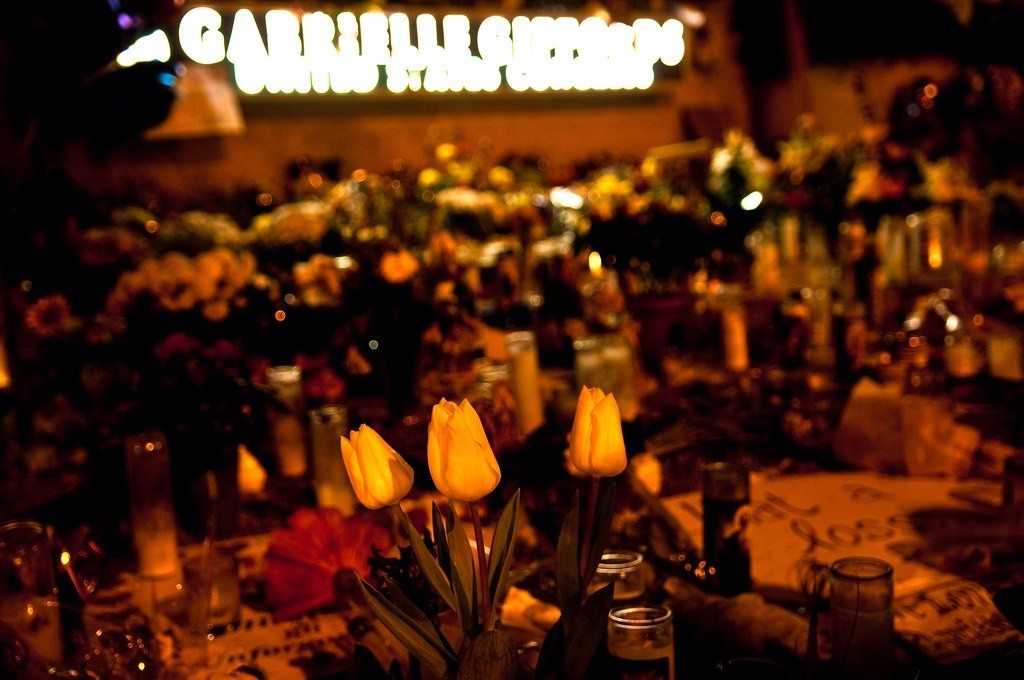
[183,551,244,637]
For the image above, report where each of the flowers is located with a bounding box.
[257,383,627,679]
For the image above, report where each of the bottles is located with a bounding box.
[587,551,646,611]
[605,602,676,680]
[310,409,357,523]
[698,462,756,597]
[509,335,547,438]
[125,429,178,580]
[826,555,896,679]
[264,365,305,484]
[1,523,63,670]
[574,196,1023,442]
